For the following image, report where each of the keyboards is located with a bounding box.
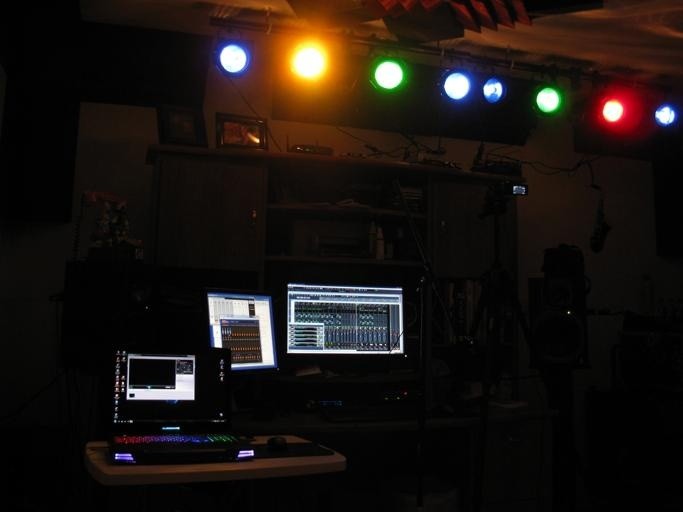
[111,433,239,454]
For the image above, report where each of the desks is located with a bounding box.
[84,430,348,510]
[146,420,523,503]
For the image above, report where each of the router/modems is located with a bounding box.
[286,133,334,156]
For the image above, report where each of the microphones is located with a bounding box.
[588,209,608,253]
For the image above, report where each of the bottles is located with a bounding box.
[639,273,656,320]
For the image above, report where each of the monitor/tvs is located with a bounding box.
[104,343,254,434]
[281,279,409,380]
[199,287,282,416]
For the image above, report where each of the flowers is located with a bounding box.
[96,202,147,260]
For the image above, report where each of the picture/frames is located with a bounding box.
[213,110,269,150]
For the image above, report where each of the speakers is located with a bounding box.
[0,73,82,224]
[652,142,683,258]
[61,261,161,365]
[528,277,592,373]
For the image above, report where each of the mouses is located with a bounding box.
[265,436,288,452]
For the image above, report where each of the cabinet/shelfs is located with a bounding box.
[146,145,523,417]
